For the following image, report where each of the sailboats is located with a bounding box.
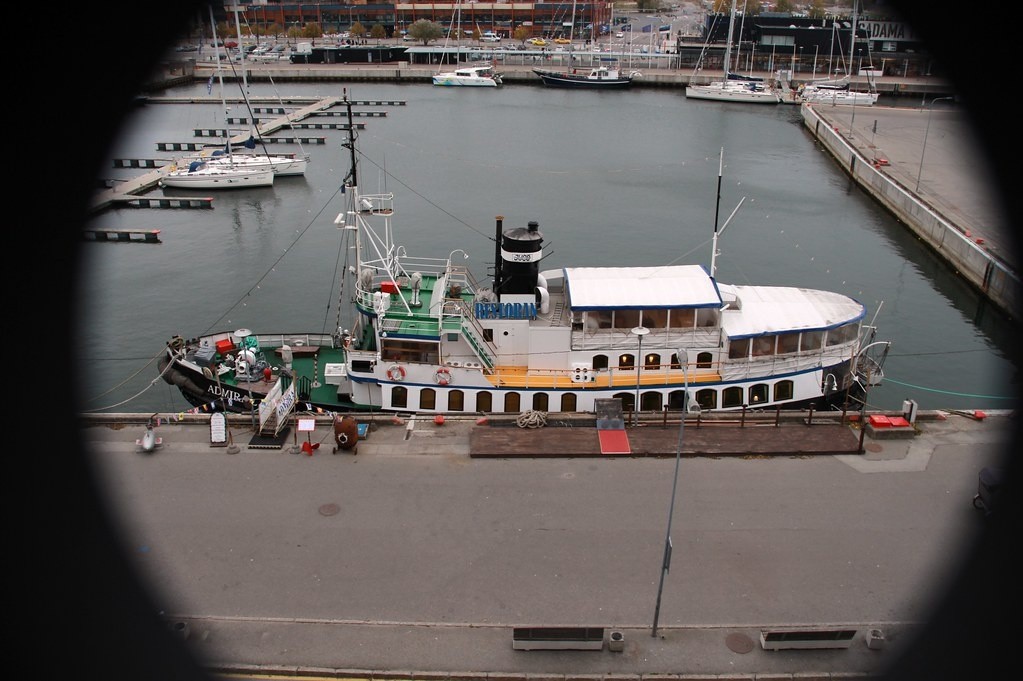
[686,0,881,107]
[533,0,635,84]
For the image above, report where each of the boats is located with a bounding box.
[156,86,890,413]
[852,353,883,386]
[159,152,314,188]
[434,58,503,89]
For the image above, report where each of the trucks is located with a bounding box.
[297,42,313,54]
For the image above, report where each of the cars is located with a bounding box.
[555,38,571,44]
[616,31,623,38]
[234,53,246,60]
[594,46,600,52]
[526,37,541,43]
[534,40,552,45]
[404,35,416,42]
[177,46,198,52]
[209,40,296,54]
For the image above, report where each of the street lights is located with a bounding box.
[344,5,357,30]
[248,5,262,47]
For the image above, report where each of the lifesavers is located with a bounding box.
[434,368,453,385]
[387,365,406,382]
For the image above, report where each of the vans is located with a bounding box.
[479,33,501,42]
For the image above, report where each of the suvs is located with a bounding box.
[493,43,526,52]
